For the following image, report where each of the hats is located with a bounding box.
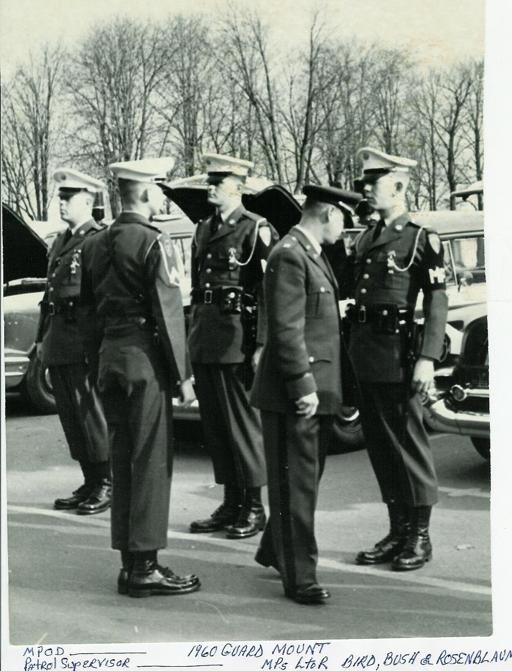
[53,148,419,230]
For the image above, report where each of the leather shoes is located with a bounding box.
[54,480,431,603]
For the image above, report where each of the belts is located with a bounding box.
[48,289,246,327]
[347,308,377,328]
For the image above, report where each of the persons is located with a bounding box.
[35,168,114,515]
[343,147,448,569]
[81,156,201,595]
[188,154,281,538]
[253,186,366,603]
